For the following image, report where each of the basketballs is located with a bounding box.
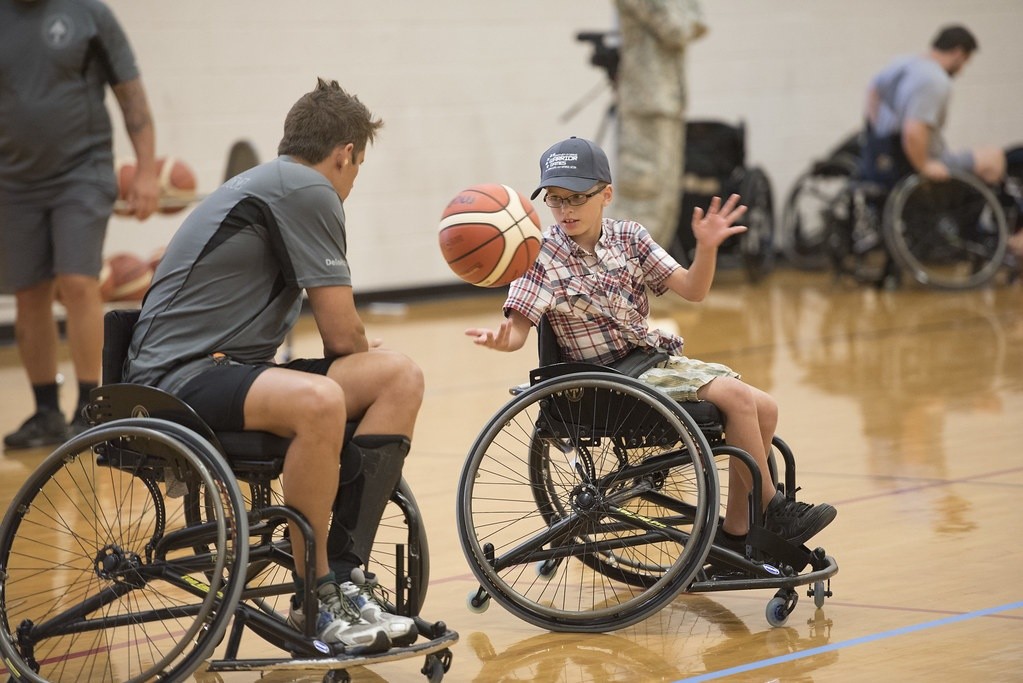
[437,184,543,288]
[53,238,168,309]
[112,156,198,217]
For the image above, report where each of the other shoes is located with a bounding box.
[61,412,91,443]
[4,412,65,448]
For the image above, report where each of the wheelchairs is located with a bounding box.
[782,131,1023,291]
[575,32,775,284]
[457,314,839,634]
[0,309,460,683]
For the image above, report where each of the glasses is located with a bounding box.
[544,185,606,208]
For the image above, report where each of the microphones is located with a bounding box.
[578,32,603,42]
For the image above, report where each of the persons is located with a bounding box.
[464,136,837,563]
[121,74,426,659]
[611,0,707,251]
[0,0,159,449]
[865,23,1012,248]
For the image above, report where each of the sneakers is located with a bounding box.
[285,580,393,654]
[707,525,780,573]
[763,486,837,547]
[340,581,418,647]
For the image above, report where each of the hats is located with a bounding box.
[531,136,612,200]
